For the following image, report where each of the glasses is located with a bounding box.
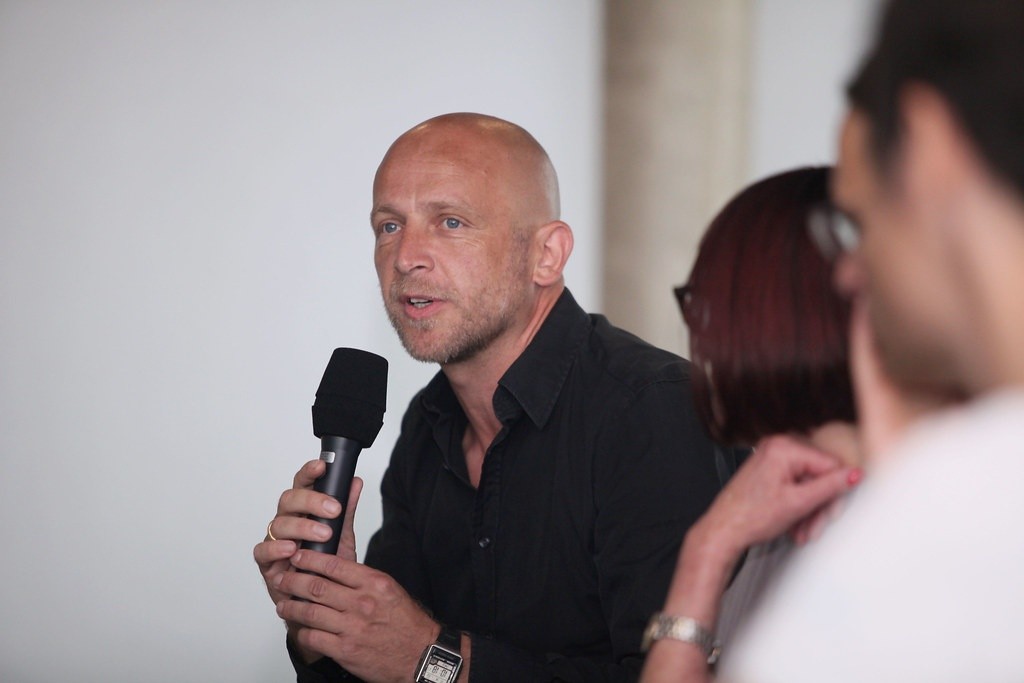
[804,187,884,265]
[673,284,690,313]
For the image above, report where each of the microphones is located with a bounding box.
[293,347,389,604]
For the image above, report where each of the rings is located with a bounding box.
[267,520,276,541]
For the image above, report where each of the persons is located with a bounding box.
[252,112,730,683]
[642,0,1024,683]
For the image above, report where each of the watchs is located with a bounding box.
[412,624,464,683]
[640,609,721,668]
